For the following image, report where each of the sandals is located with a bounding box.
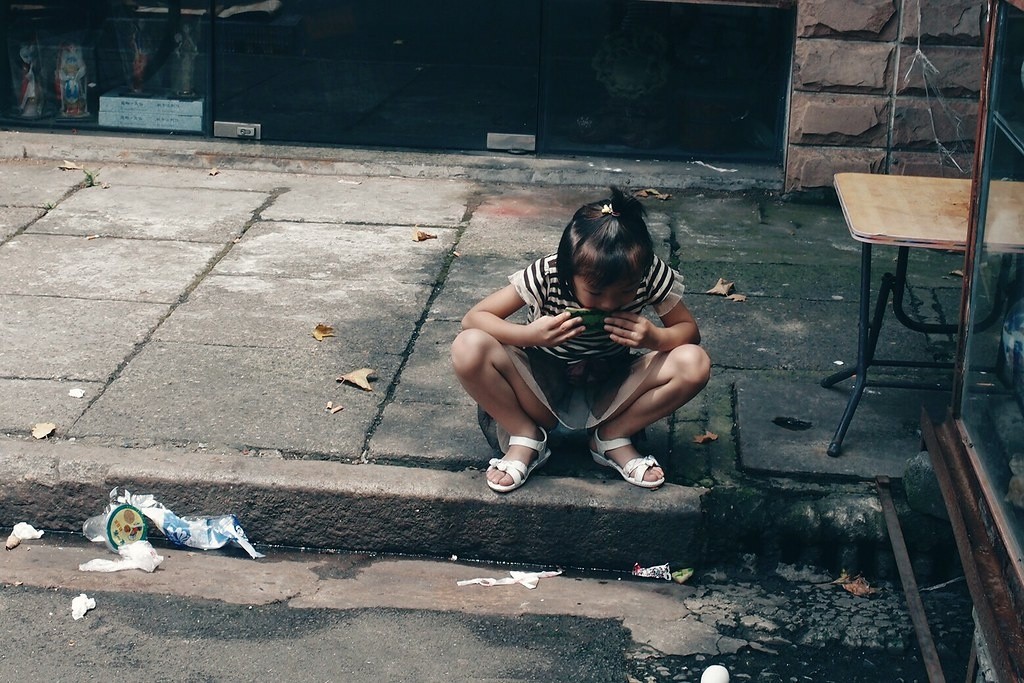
[486,426,552,492]
[590,427,665,488]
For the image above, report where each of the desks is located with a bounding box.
[821,173,973,456]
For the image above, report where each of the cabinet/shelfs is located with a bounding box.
[875,0,1024,683]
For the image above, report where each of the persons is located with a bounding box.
[131,14,155,88]
[174,22,201,98]
[58,38,89,117]
[449,184,711,494]
[19,38,46,117]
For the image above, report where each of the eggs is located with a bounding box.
[700,664,729,683]
